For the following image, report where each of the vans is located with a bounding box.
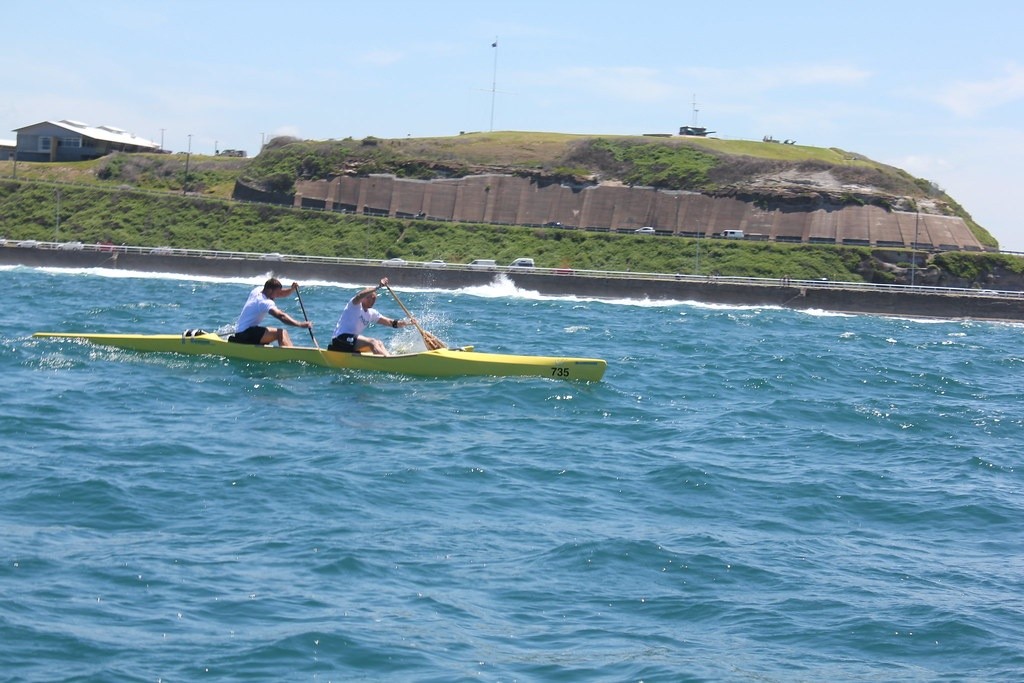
[718,229,745,239]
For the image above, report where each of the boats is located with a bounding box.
[29,329,611,387]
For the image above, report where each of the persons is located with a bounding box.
[329,276,422,358]
[229,277,314,349]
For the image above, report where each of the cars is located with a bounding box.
[635,225,654,234]
[0,237,573,275]
[543,221,562,227]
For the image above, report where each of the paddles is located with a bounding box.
[295,284,320,349]
[386,283,446,351]
